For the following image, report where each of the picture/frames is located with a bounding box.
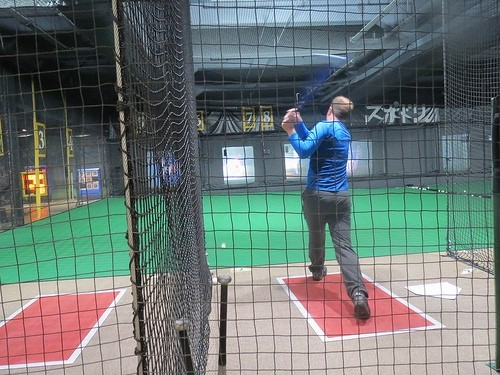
[196,106,274,131]
[36,122,76,157]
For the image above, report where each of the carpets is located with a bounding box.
[0,288,128,370]
[275,270,448,343]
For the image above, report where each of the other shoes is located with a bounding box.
[313,267,327,281]
[352,292,371,319]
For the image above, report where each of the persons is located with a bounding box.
[281,96,371,319]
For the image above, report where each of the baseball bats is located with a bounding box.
[215,275,232,375]
[175,321,196,372]
